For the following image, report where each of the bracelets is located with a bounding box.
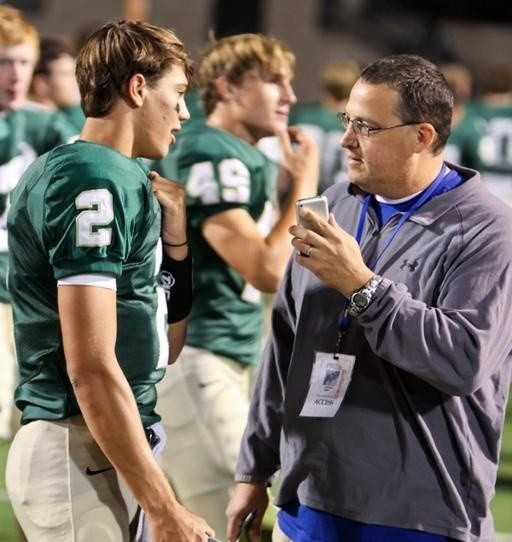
[162,239,187,247]
[159,243,193,323]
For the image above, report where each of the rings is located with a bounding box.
[306,247,313,255]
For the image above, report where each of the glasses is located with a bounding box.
[337,112,441,138]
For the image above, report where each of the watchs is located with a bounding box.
[349,275,383,317]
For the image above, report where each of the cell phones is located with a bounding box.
[295,196,330,257]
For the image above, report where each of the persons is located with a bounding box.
[121,4,360,209]
[1,6,87,441]
[1,19,218,542]
[437,61,512,207]
[144,29,323,542]
[219,50,512,540]
